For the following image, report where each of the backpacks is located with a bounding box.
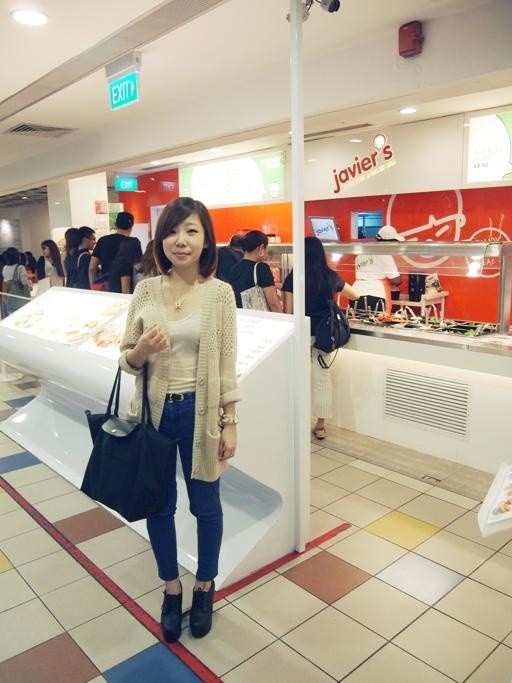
[313,299,350,353]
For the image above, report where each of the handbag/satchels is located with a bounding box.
[5,281,31,315]
[241,286,271,311]
[81,411,182,522]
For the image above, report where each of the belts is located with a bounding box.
[164,394,186,401]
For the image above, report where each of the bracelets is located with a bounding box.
[220,413,237,425]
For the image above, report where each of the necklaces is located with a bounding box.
[167,272,199,310]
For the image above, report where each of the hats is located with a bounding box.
[117,212,133,223]
[376,225,406,242]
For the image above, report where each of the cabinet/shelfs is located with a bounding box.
[264,243,511,344]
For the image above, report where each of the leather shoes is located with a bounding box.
[190,580,215,638]
[161,580,182,643]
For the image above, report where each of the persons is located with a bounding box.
[69,226,97,289]
[0,247,37,318]
[353,225,405,316]
[37,239,65,286]
[64,228,80,287]
[88,212,142,294]
[280,236,359,441]
[214,235,244,279]
[132,240,162,293]
[230,231,282,313]
[120,199,240,642]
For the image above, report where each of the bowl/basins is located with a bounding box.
[392,289,402,299]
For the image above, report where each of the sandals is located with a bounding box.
[313,426,326,440]
